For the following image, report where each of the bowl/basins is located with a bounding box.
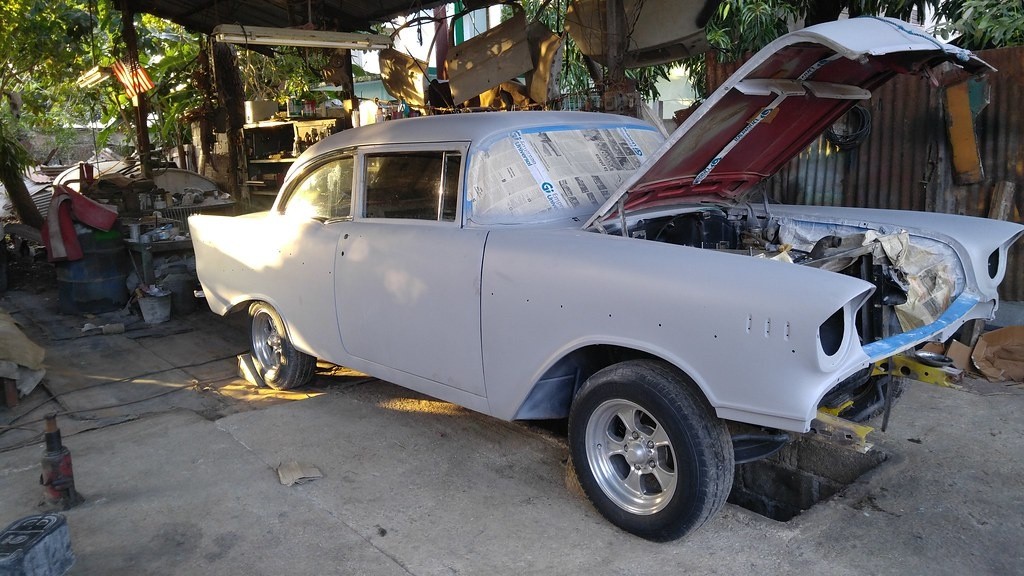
[914,350,954,368]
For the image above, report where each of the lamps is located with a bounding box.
[211,0,394,50]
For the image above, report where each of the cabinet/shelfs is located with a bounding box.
[241,119,302,197]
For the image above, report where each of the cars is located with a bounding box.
[187,15,1024,543]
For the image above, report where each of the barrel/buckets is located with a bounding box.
[136,291,172,323]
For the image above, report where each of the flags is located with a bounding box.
[111,54,155,98]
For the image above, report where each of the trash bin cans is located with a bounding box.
[137,287,172,324]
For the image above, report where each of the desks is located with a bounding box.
[122,238,193,285]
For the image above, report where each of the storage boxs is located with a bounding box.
[970,324,1024,383]
